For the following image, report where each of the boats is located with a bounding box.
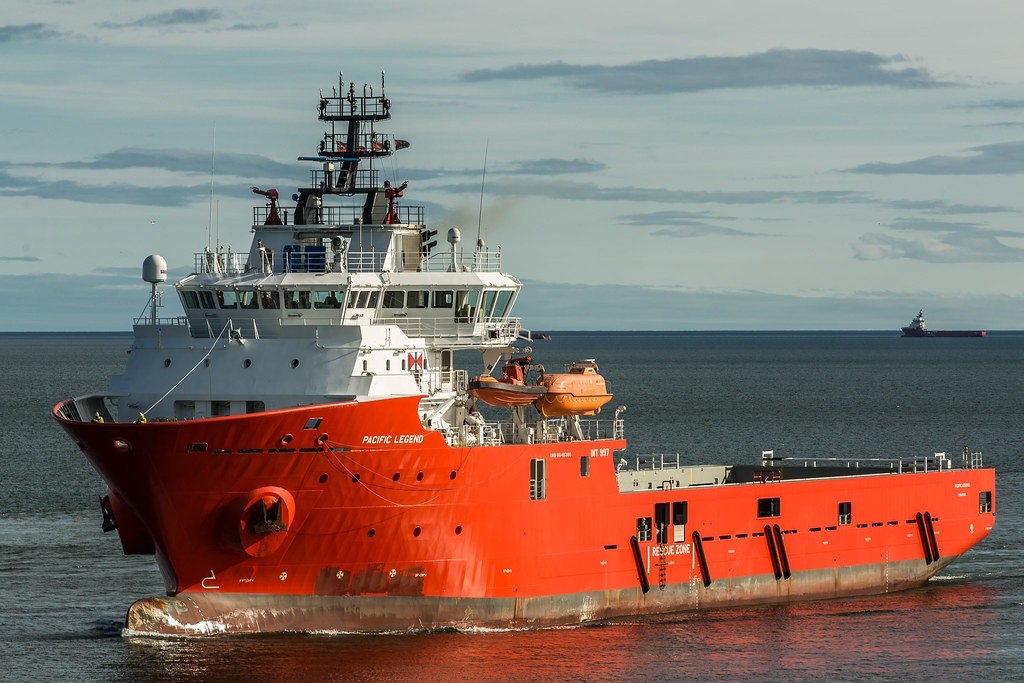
[47,67,997,643]
[467,345,547,409]
[534,358,613,419]
[895,311,988,339]
